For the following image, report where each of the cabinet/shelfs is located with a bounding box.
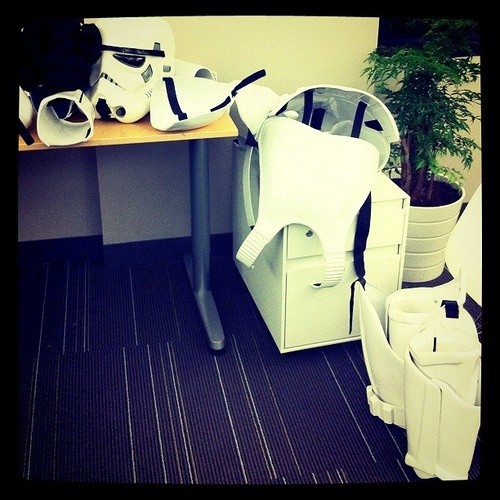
[227,139,415,356]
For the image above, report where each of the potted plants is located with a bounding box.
[357,18,482,285]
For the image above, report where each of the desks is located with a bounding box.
[18,110,242,354]
